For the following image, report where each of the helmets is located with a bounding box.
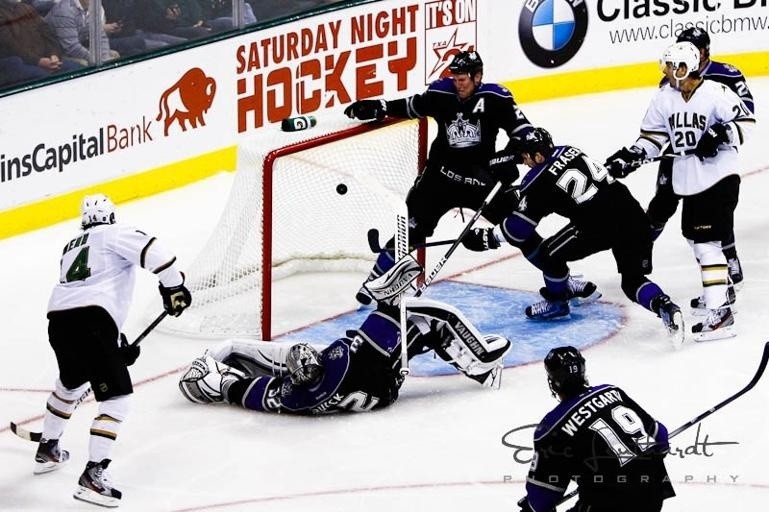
[449,51,483,80]
[518,128,551,155]
[81,195,116,226]
[663,27,710,72]
[544,345,585,397]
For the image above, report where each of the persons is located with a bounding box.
[35,194,191,502]
[641,27,755,284]
[515,346,676,511]
[602,40,757,336]
[460,128,682,343]
[343,51,597,306]
[178,293,513,416]
[0,1,322,89]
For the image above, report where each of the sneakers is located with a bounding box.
[35,439,69,463]
[356,287,373,305]
[526,278,596,319]
[79,459,121,499]
[660,257,742,333]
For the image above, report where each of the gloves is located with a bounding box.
[694,123,730,161]
[119,333,140,366]
[604,146,647,178]
[159,271,191,316]
[462,228,501,251]
[344,100,387,122]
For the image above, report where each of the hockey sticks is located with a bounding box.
[368,229,461,252]
[554,343,769,506]
[11,310,168,442]
[395,215,411,378]
